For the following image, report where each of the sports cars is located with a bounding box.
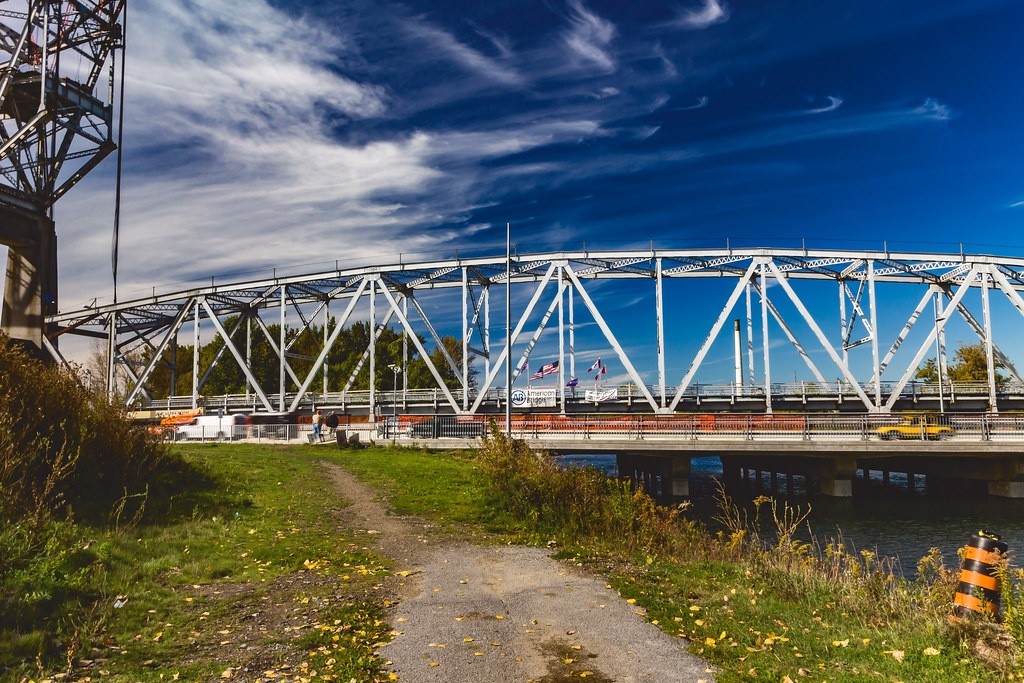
[876,417,955,441]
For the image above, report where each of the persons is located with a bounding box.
[325,411,339,439]
[311,410,326,434]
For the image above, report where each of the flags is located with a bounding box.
[529,360,559,380]
[567,377,579,386]
[587,356,601,373]
[595,364,606,380]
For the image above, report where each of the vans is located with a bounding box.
[176,414,248,441]
